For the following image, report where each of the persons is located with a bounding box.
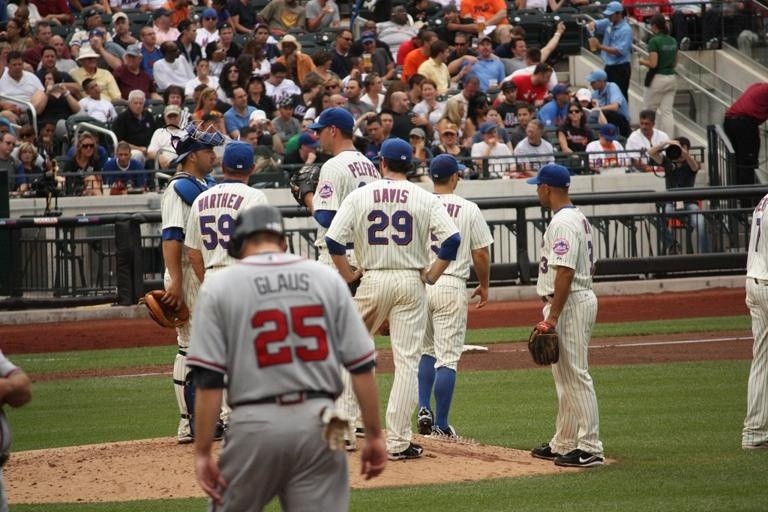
[741,194,768,449]
[1,1,681,197]
[650,136,712,254]
[185,202,387,511]
[325,137,461,459]
[418,154,494,438]
[1,351,33,512]
[722,82,768,206]
[163,120,229,442]
[527,164,604,466]
[670,1,767,58]
[185,142,268,443]
[306,107,381,435]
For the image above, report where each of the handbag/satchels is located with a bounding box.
[643,70,657,88]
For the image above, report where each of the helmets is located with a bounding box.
[225,205,287,259]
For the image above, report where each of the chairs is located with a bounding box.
[0,0,766,294]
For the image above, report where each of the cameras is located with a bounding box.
[32,175,58,195]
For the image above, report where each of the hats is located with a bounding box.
[222,141,254,171]
[378,135,414,163]
[69,0,625,155]
[526,162,570,188]
[429,153,467,178]
[174,134,213,164]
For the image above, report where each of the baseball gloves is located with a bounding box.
[528,322,559,366]
[289,164,321,206]
[145,290,189,327]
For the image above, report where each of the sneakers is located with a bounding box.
[554,448,605,468]
[386,442,425,462]
[529,442,562,461]
[741,440,768,450]
[354,427,365,438]
[416,406,434,436]
[177,418,225,444]
[343,440,357,452]
[432,424,460,440]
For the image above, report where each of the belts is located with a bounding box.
[541,293,556,304]
[227,388,337,411]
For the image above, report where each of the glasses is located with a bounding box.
[1,139,16,147]
